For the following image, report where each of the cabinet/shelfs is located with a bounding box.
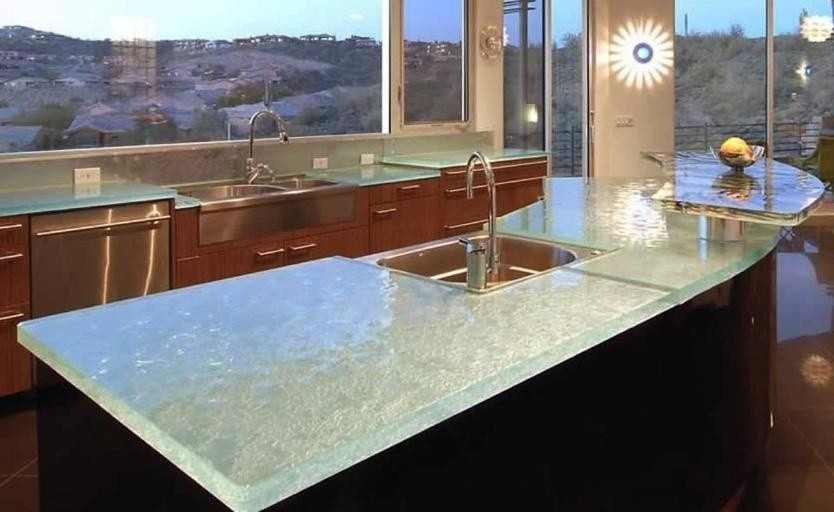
[1,214,32,396]
[440,156,549,239]
[172,186,370,288]
[369,177,440,259]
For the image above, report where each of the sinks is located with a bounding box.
[371,229,579,289]
[210,171,339,191]
[160,184,287,204]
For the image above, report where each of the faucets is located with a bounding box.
[244,109,290,182]
[464,148,498,280]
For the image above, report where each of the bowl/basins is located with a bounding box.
[709,144,765,171]
[709,171,763,196]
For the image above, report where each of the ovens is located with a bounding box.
[28,198,170,319]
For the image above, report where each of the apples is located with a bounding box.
[721,137,747,154]
